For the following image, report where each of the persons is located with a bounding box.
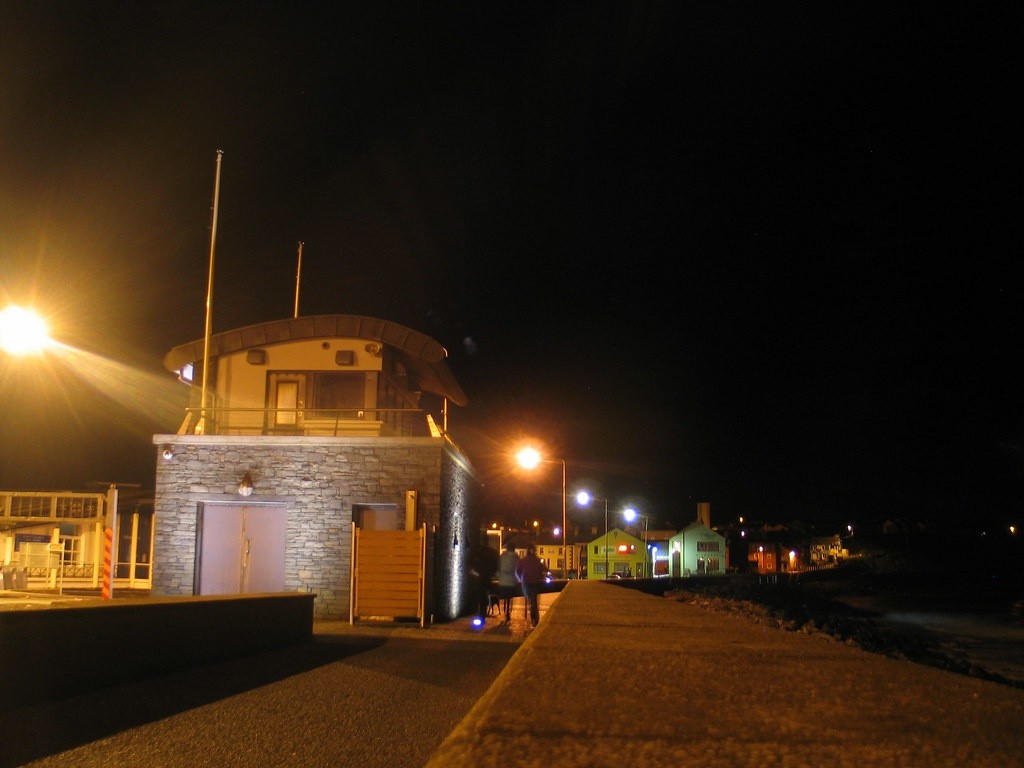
[514,542,546,627]
[496,540,520,621]
[467,534,499,626]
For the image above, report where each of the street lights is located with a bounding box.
[621,510,650,577]
[576,490,608,579]
[519,449,567,579]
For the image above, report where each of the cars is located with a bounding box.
[605,571,634,578]
